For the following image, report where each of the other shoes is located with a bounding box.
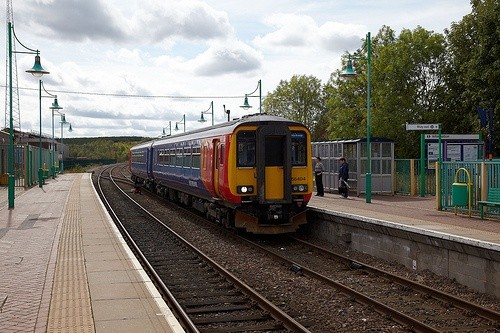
[321,194,324,196]
[339,196,347,199]
[315,194,321,196]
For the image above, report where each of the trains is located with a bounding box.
[128,113,314,241]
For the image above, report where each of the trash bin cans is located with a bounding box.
[52,166,59,172]
[0,173,9,187]
[38,169,49,179]
[452,181,474,207]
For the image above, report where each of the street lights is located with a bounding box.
[7,22,50,208]
[338,31,371,203]
[173,114,185,132]
[197,101,214,125]
[241,80,262,113]
[162,121,171,136]
[39,80,63,188]
[51,103,66,179]
[60,115,74,175]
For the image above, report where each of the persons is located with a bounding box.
[338,158,349,199]
[314,157,325,197]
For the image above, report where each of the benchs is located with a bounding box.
[477,199,500,220]
